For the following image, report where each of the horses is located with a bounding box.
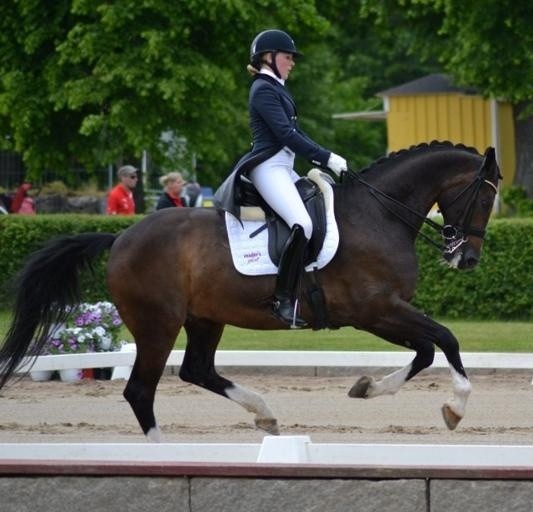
[0,140,506,444]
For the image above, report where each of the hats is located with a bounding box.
[117,165,139,177]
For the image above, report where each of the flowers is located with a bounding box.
[26,300,126,354]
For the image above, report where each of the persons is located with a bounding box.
[209,27,348,327]
[153,170,188,211]
[11,182,37,213]
[105,165,140,216]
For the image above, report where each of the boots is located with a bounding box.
[266,222,311,330]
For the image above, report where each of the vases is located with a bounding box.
[29,366,110,382]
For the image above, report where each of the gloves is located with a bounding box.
[327,151,349,177]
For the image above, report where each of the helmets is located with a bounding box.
[250,29,303,62]
